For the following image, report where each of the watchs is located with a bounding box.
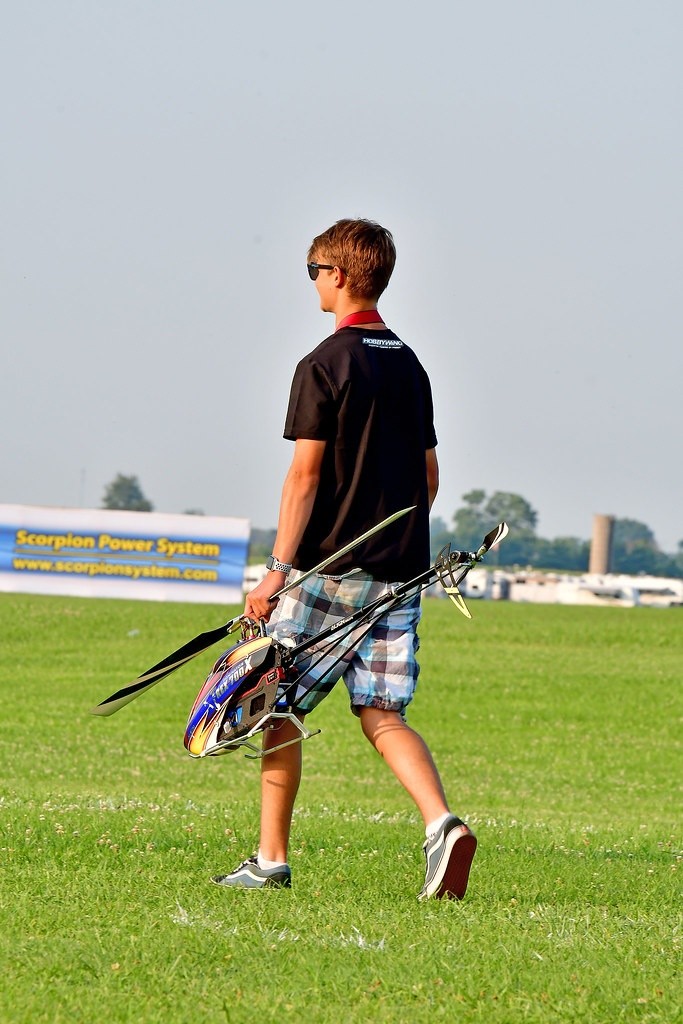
[266,554,293,574]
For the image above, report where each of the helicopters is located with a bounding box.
[93,508,509,761]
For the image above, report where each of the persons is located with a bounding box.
[207,216,478,904]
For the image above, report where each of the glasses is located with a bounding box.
[307,262,351,280]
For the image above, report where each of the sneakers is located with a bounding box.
[209,852,291,892]
[417,816,477,900]
